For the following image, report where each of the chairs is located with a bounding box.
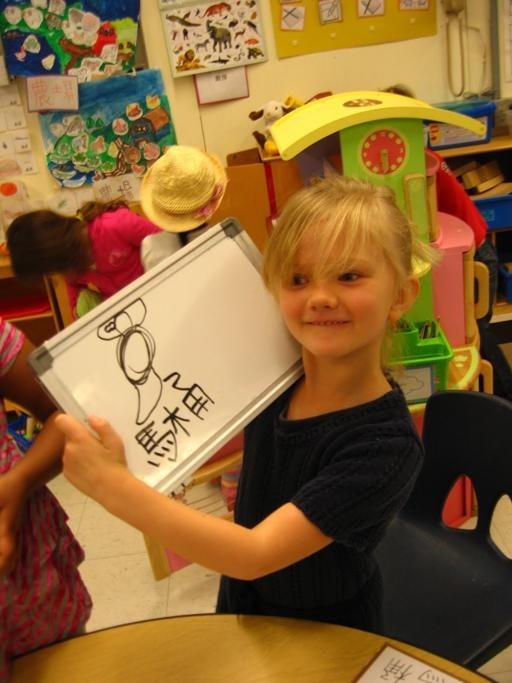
[368,388,511,672]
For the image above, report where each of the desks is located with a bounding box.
[10,613,498,682]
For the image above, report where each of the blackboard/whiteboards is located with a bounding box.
[26,218,303,494]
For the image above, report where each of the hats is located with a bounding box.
[140,142,231,233]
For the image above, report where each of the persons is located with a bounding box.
[0,317,94,660]
[52,174,428,634]
[4,199,182,321]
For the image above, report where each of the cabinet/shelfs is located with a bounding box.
[0,256,62,344]
[427,134,512,325]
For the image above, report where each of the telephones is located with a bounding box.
[441,0,466,16]
